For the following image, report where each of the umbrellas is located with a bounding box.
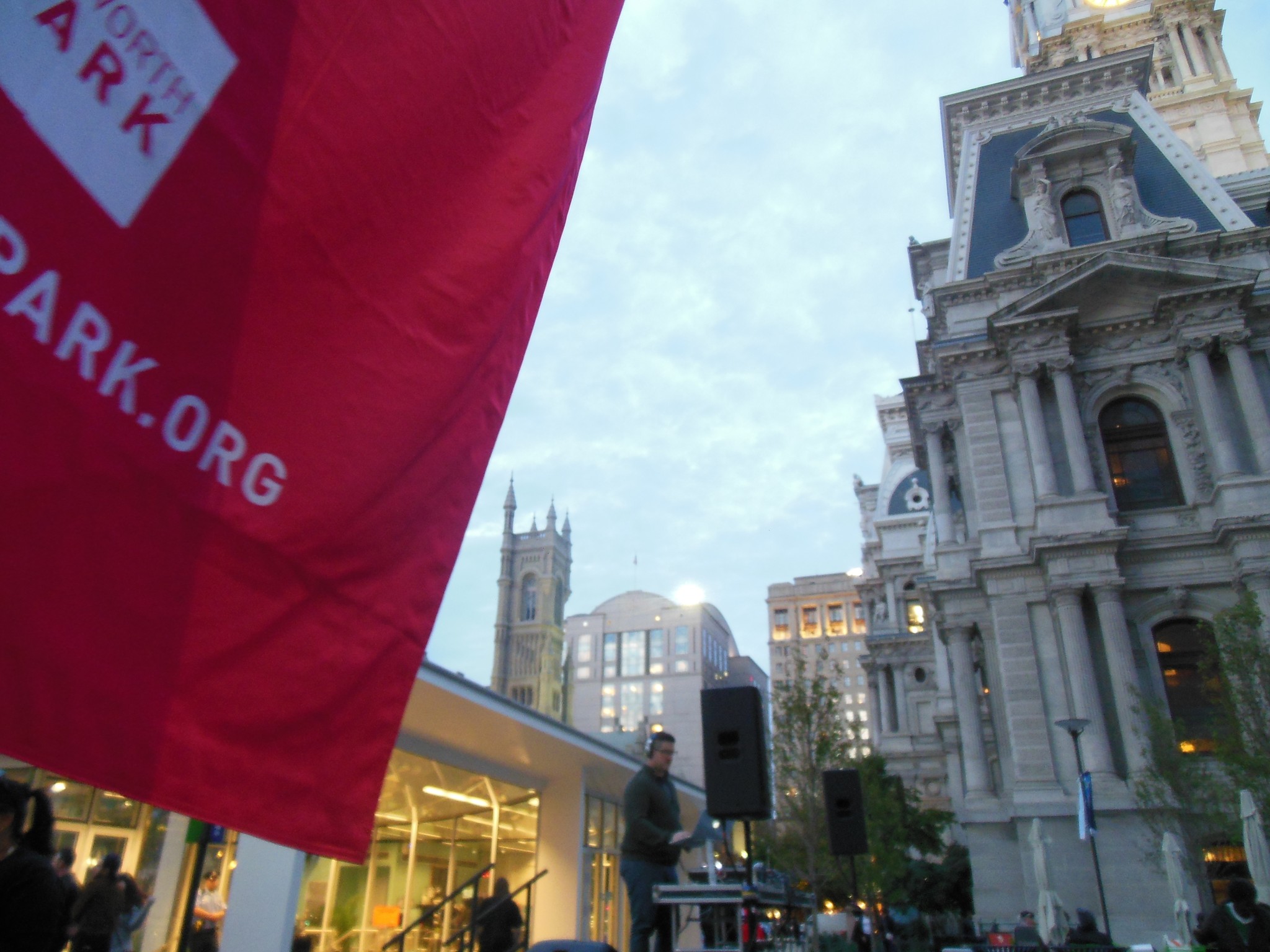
[1162,832,1199,952]
[1238,789,1270,906]
[1028,818,1070,952]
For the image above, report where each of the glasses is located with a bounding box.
[651,747,675,756]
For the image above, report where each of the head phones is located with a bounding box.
[644,732,657,757]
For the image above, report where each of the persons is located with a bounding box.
[473,877,524,951]
[0,776,226,952]
[618,731,694,952]
[1013,876,1270,952]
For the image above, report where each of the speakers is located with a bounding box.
[821,769,866,858]
[699,685,770,821]
